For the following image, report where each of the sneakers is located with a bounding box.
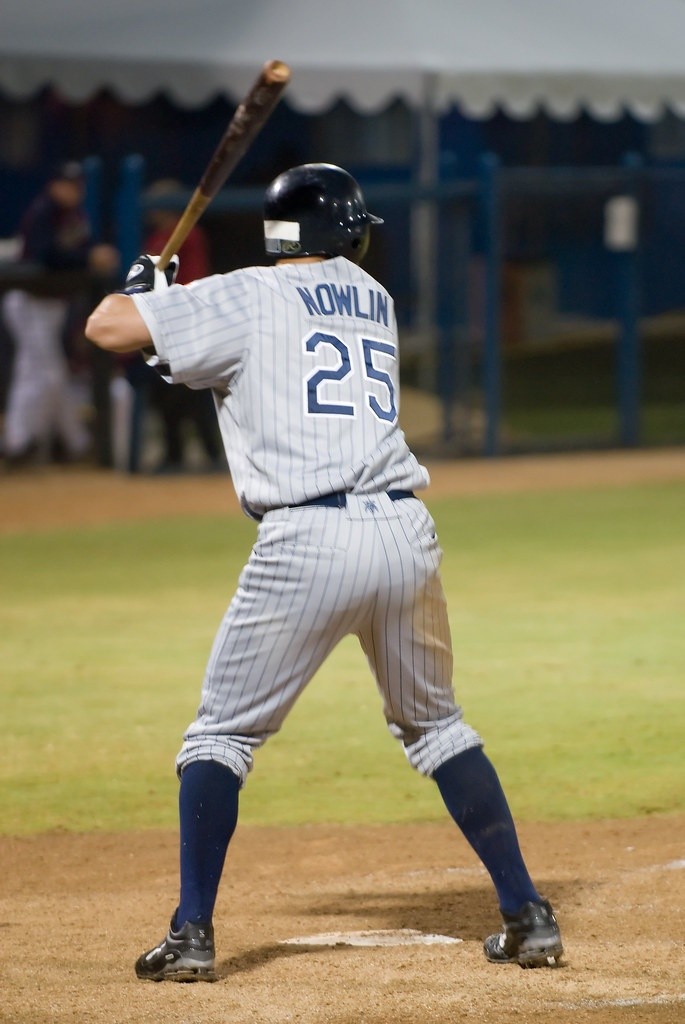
[483,898,569,969]
[134,907,219,983]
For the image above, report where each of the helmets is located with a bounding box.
[262,162,384,265]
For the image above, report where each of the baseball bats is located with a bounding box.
[155,55,296,271]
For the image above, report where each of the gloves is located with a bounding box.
[121,253,180,295]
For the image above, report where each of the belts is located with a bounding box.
[291,489,416,507]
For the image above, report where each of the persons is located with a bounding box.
[1,161,222,475]
[85,163,564,983]
